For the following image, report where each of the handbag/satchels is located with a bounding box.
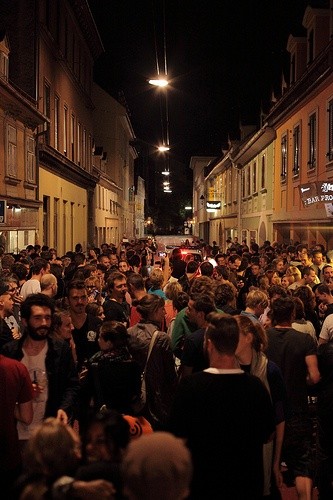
[132,374,147,412]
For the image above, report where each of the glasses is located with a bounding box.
[7,287,18,292]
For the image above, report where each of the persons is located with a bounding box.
[0,233,333,500]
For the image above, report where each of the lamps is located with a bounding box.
[149,44,172,193]
